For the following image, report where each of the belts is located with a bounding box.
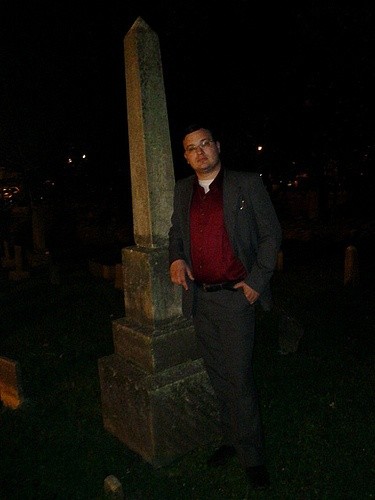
[194,277,241,294]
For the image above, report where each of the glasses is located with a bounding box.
[183,137,215,153]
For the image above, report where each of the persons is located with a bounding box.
[168,126,282,490]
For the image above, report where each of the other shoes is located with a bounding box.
[246,453,272,493]
[207,444,237,467]
